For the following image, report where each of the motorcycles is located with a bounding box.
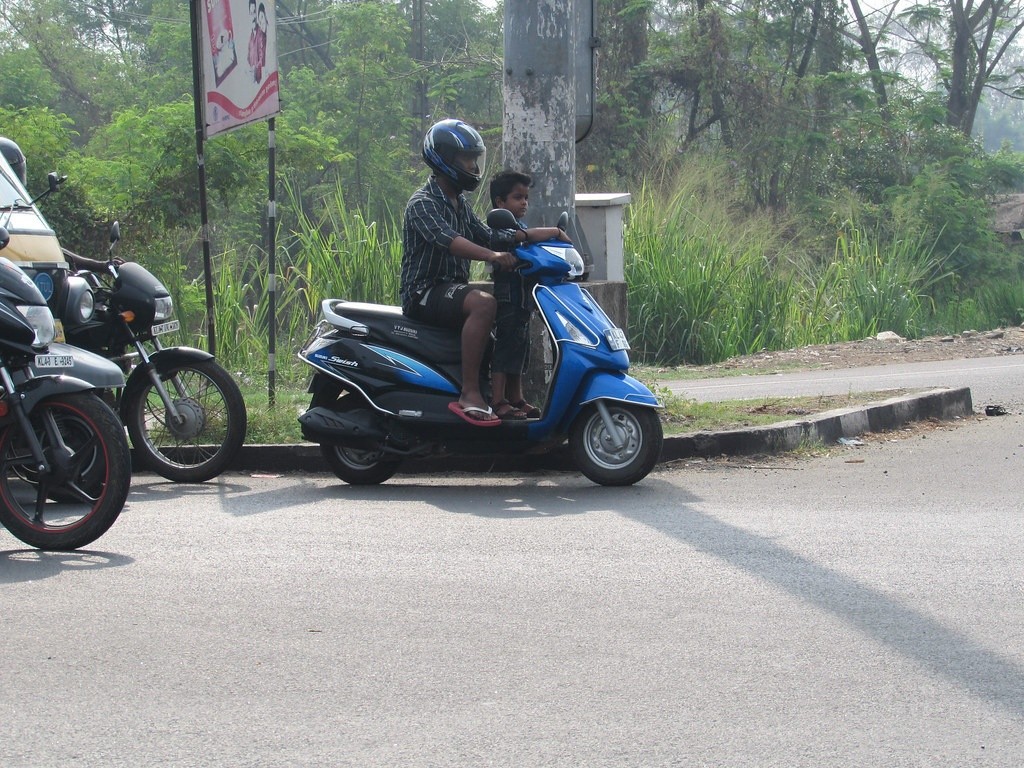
[0,152,247,551]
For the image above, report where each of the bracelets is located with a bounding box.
[556,227,561,241]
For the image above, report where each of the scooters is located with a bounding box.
[298,208,665,487]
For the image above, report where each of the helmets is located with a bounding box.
[421,119,486,192]
[0,137,26,186]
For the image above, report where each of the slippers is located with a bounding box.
[448,402,501,427]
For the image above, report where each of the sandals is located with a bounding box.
[489,399,527,420]
[509,399,541,417]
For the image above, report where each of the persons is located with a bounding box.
[399,118,502,425]
[246,0,279,86]
[486,168,572,419]
[0,136,125,275]
